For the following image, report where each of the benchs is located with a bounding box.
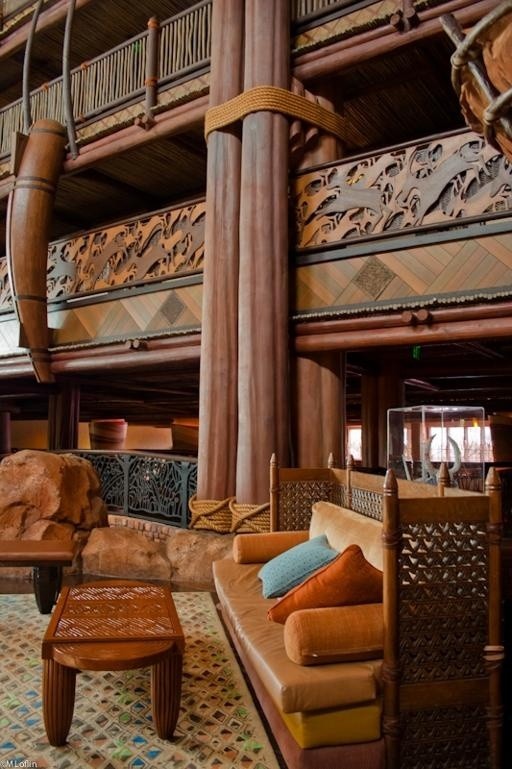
[0,539,78,615]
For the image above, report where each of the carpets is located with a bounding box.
[0,590,281,768]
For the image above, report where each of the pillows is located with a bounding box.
[256,534,383,625]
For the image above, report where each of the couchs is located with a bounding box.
[210,451,503,769]
